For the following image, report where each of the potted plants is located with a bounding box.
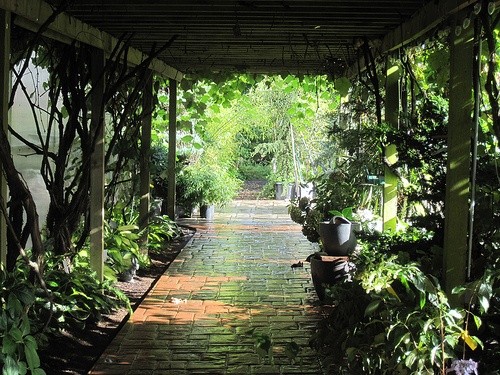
[311,253,353,299]
[313,210,361,256]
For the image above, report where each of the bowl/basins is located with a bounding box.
[329,216,352,224]
